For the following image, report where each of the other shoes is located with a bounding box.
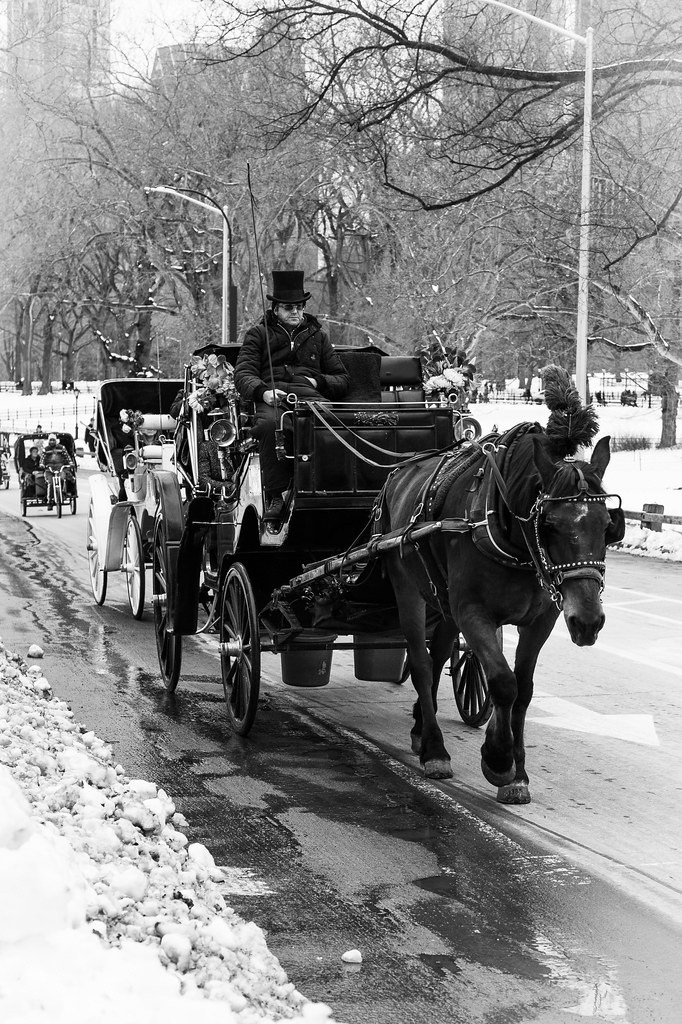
[48,505,53,510]
[21,495,34,501]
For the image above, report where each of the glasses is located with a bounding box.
[279,303,304,310]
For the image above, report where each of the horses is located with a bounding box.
[371,431,611,804]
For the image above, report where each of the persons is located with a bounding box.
[171,389,194,421]
[69,378,74,391]
[62,379,68,394]
[23,447,41,498]
[85,418,97,459]
[234,271,352,524]
[34,425,44,434]
[41,433,76,511]
[15,377,24,390]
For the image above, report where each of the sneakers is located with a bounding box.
[262,498,284,522]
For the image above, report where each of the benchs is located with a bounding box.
[114,414,179,514]
[22,458,75,492]
[198,355,423,531]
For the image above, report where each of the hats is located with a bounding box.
[90,417,96,421]
[266,270,312,304]
[48,434,57,447]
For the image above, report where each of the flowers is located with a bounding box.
[188,352,238,414]
[119,408,148,445]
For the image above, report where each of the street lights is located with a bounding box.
[143,185,231,345]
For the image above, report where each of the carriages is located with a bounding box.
[80,344,626,804]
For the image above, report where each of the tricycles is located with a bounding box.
[13,432,80,519]
